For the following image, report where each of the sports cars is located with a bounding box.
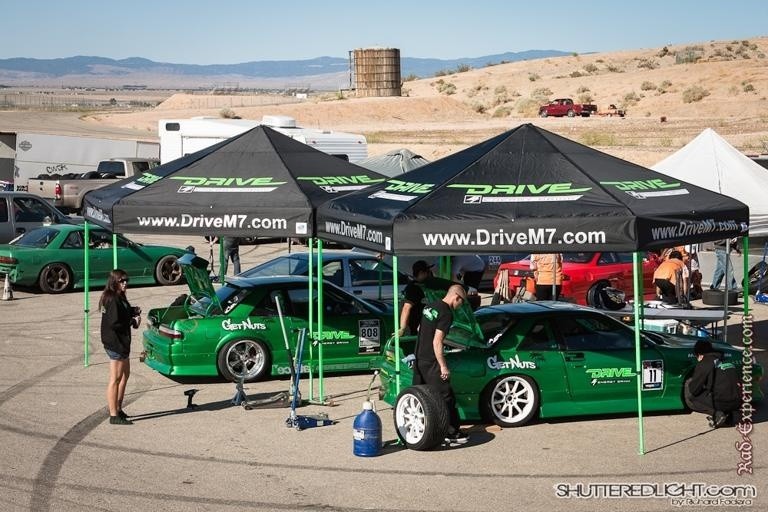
[138,254,416,384]
[492,247,669,308]
[1,225,192,294]
[233,250,482,327]
[373,298,765,425]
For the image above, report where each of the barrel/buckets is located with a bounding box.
[689,324,708,337]
[351,401,383,457]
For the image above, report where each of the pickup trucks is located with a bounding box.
[539,99,597,119]
[27,157,161,218]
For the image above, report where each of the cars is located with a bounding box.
[466,251,516,291]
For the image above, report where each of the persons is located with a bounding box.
[710,237,744,297]
[209,236,240,276]
[688,342,742,428]
[415,284,470,442]
[530,254,562,300]
[392,261,429,336]
[652,251,690,305]
[98,270,141,424]
[452,255,485,290]
[660,244,703,299]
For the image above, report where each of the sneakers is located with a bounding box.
[706,414,732,428]
[444,432,471,443]
[119,410,129,418]
[109,416,132,424]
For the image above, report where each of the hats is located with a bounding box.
[412,260,435,273]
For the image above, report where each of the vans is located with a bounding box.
[1,191,101,245]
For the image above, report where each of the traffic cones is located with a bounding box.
[2,273,15,301]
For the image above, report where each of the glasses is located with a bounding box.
[456,292,465,303]
[117,278,128,283]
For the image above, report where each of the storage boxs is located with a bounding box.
[639,318,679,335]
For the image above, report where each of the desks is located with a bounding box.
[592,303,729,345]
